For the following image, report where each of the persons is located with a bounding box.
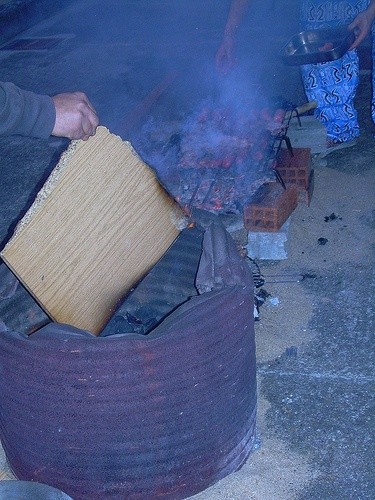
[0,81,100,142]
[212,0,375,144]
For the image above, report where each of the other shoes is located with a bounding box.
[319,137,359,158]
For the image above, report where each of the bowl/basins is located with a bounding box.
[278,24,356,66]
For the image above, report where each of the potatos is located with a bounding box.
[179,106,286,169]
